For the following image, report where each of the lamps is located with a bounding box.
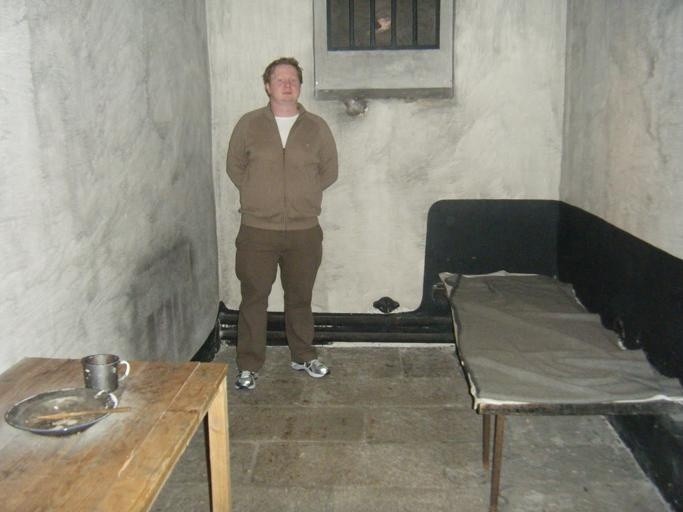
[339,93,369,119]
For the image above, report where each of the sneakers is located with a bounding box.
[291,359,332,379]
[234,370,259,390]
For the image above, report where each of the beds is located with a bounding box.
[434,267,683,511]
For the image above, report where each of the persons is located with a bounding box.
[225,58,338,392]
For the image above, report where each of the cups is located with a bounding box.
[80,354,129,392]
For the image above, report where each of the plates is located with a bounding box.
[5,386,116,438]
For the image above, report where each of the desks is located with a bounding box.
[0,351,234,512]
